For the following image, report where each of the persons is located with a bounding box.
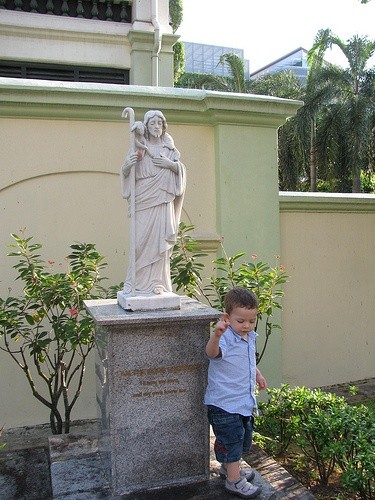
[207,288,267,496]
[121,106,187,311]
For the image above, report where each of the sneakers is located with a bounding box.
[224,476,261,498]
[219,464,256,481]
